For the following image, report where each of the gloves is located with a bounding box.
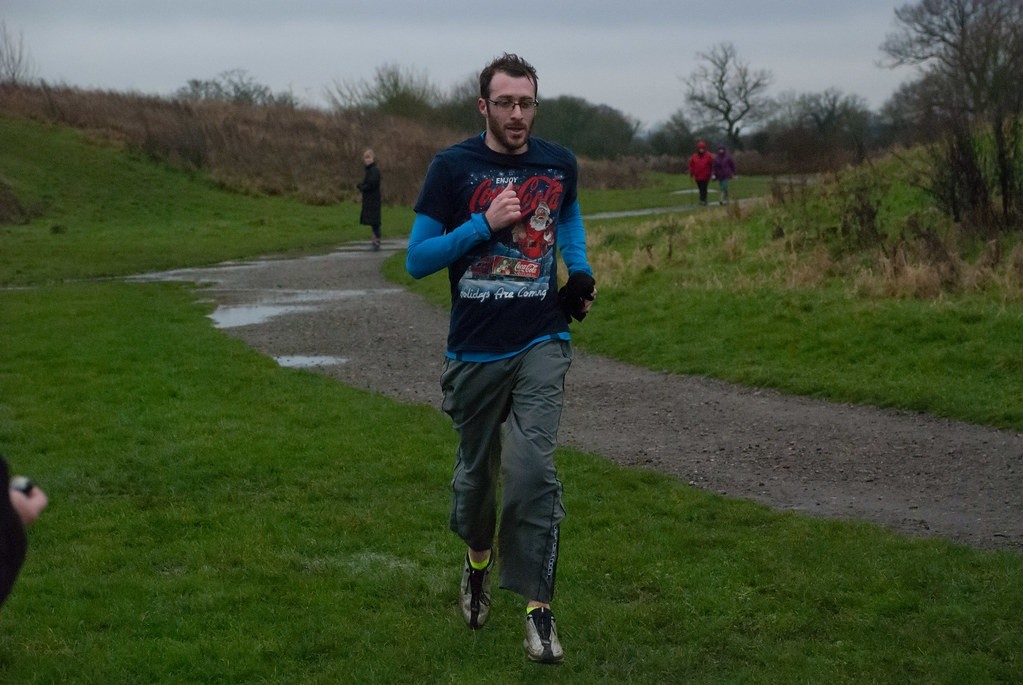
[557,271,597,322]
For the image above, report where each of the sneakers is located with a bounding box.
[521,607,566,662]
[460,547,497,629]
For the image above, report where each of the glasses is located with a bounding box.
[485,95,539,113]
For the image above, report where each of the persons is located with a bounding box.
[0,456,48,610]
[687,141,713,205]
[406,54,598,664]
[710,144,736,204]
[356,148,381,249]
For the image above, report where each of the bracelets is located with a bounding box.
[482,212,493,233]
